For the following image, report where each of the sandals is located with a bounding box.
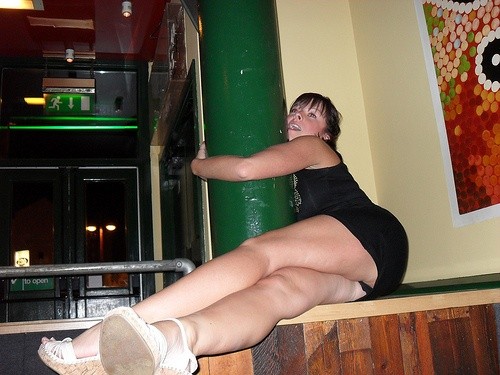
[38,337,107,375]
[98,306,198,375]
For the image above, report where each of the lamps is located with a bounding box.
[65,48,75,63]
[121,1,132,18]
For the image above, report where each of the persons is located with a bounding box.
[38,93,409,375]
